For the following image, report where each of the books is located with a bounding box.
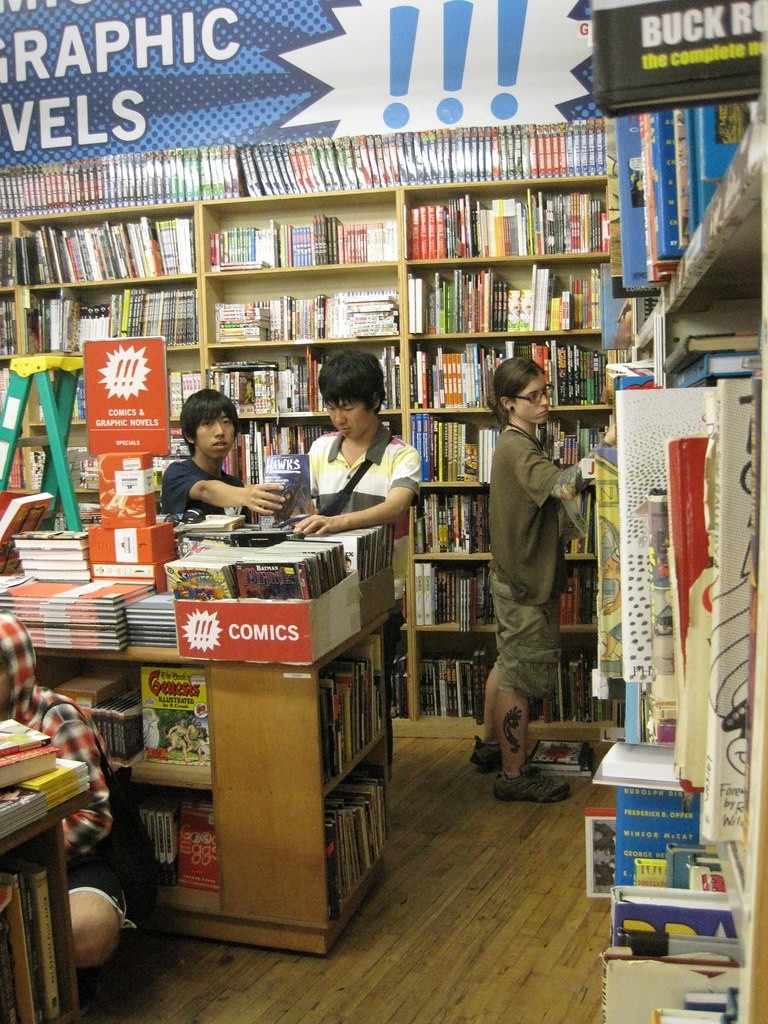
[589,0,768,1024]
[0,114,606,1024]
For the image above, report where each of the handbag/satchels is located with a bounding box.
[41,699,159,927]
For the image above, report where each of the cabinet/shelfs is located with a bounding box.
[0,788,95,1024]
[30,609,390,956]
[1,0,768,1023]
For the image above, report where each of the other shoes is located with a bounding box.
[76,965,99,1016]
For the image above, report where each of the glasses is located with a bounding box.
[502,384,554,403]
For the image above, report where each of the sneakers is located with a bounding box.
[493,759,570,804]
[469,735,503,770]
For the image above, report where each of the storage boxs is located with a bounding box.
[88,521,175,563]
[359,564,396,628]
[90,557,179,595]
[173,568,362,665]
[97,450,157,528]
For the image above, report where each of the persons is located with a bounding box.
[160,389,286,517]
[470,358,617,803]
[0,608,135,972]
[294,351,422,781]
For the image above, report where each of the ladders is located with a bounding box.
[0,355,86,533]
